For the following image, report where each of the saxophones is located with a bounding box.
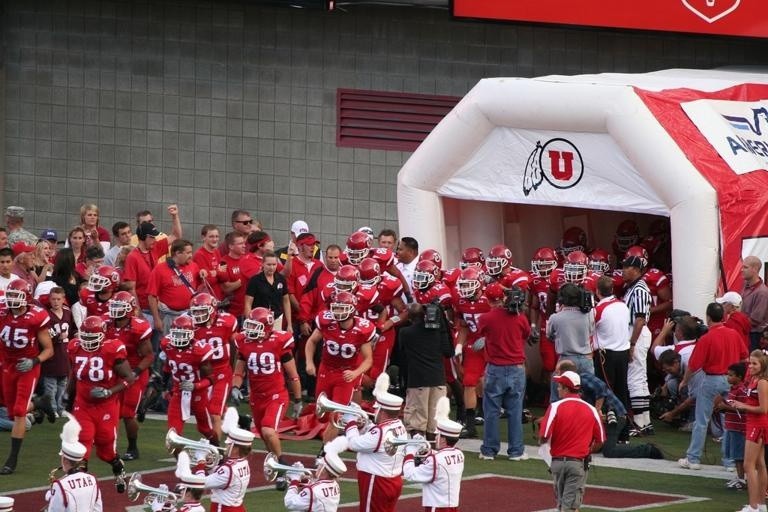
[39,466,62,512]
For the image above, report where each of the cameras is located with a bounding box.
[605,408,619,428]
[218,298,232,310]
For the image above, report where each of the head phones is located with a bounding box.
[137,222,146,241]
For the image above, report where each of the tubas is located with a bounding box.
[384,428,436,458]
[165,427,228,471]
[127,473,183,511]
[264,450,317,487]
[314,392,374,428]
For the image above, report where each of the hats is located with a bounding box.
[316,451,347,476]
[136,222,162,237]
[11,242,38,254]
[40,230,58,241]
[291,221,309,237]
[177,475,206,488]
[0,495,14,512]
[551,371,581,389]
[296,233,320,245]
[5,206,27,220]
[434,419,463,437]
[716,292,743,308]
[372,392,403,411]
[59,440,86,462]
[225,427,255,446]
[356,227,374,240]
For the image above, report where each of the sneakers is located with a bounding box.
[455,409,531,436]
[29,393,69,423]
[509,452,529,461]
[112,457,126,493]
[724,466,768,512]
[239,415,253,427]
[678,458,701,470]
[121,449,139,460]
[0,460,16,473]
[647,442,665,459]
[618,423,655,444]
[148,368,163,386]
[479,452,494,460]
[276,461,290,490]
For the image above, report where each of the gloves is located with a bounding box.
[406,433,430,454]
[194,439,209,462]
[290,462,305,481]
[91,388,111,398]
[343,402,361,423]
[472,337,485,352]
[369,330,380,348]
[291,398,303,420]
[178,380,194,391]
[230,387,244,406]
[453,344,463,364]
[151,498,164,512]
[16,356,41,372]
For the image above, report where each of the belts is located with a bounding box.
[552,457,583,462]
[424,507,459,512]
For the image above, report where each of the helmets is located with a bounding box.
[79,316,107,353]
[170,316,196,348]
[531,221,648,285]
[87,266,122,293]
[412,250,441,289]
[244,308,274,340]
[108,291,136,321]
[189,293,217,324]
[457,245,512,299]
[5,281,33,310]
[330,232,381,321]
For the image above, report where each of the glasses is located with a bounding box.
[233,220,253,225]
[361,230,373,234]
